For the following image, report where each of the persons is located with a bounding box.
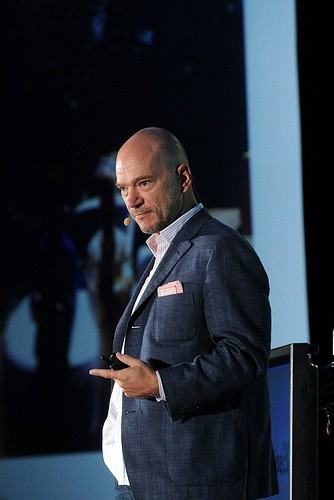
[88,125,281,500]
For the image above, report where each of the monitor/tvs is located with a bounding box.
[256,343,319,500]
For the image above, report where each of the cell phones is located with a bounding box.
[100,352,130,371]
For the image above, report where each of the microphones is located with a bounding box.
[123,215,131,226]
